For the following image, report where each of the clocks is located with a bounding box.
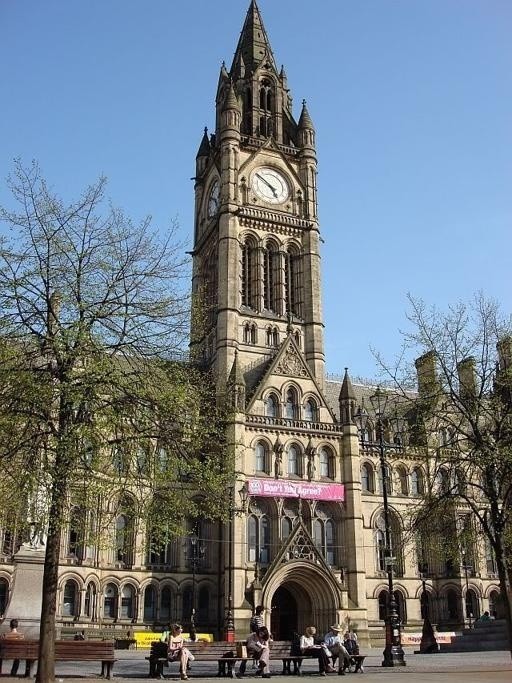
[249,163,295,206]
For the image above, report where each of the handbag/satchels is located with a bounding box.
[183,647,195,661]
[323,646,332,657]
[345,640,359,655]
[237,641,248,658]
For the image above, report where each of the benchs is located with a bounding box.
[145,640,368,679]
[0,639,118,680]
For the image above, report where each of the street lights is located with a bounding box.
[414,507,440,653]
[213,469,252,643]
[459,545,475,631]
[349,383,411,668]
[183,530,208,631]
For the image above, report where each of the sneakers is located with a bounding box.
[181,665,192,680]
[258,660,270,678]
[319,657,355,676]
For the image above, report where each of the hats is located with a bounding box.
[306,627,316,637]
[329,624,343,632]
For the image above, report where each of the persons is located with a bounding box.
[246,626,272,678]
[0,618,26,676]
[166,624,191,682]
[300,625,337,677]
[322,623,360,676]
[479,609,490,621]
[189,607,198,642]
[488,610,497,620]
[249,605,265,669]
